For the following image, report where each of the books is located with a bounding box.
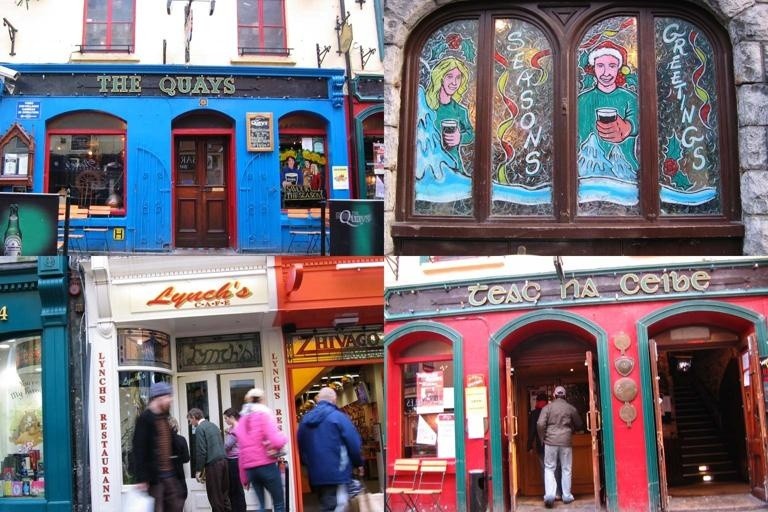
[339,401,365,437]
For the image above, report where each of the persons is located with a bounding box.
[297,387,364,512]
[309,164,324,190]
[527,392,562,500]
[280,156,303,207]
[223,408,248,511]
[302,160,311,186]
[423,57,472,177]
[187,407,232,511]
[576,41,638,171]
[239,388,290,511]
[537,386,583,507]
[132,381,188,511]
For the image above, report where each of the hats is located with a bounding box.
[149,381,174,400]
[553,385,568,397]
[588,42,631,75]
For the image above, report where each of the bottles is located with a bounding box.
[2,204,23,256]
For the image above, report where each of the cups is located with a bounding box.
[595,108,619,123]
[441,119,458,151]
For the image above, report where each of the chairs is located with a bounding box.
[287,209,331,256]
[57,203,111,256]
[402,459,449,511]
[385,457,420,511]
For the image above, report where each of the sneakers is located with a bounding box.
[545,499,554,508]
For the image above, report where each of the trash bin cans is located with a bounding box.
[468,469,488,511]
